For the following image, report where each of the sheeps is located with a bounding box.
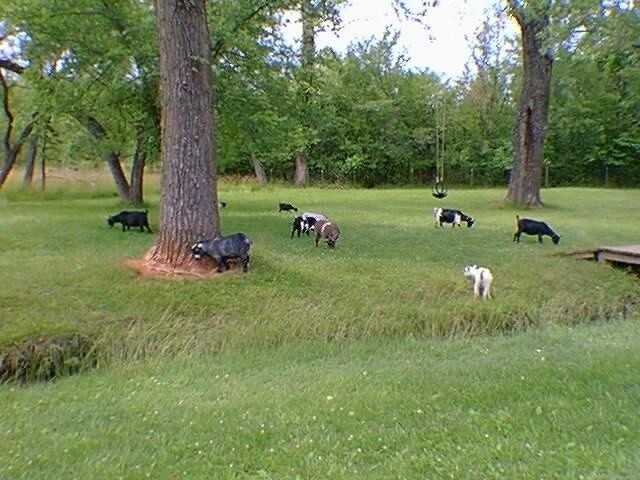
[461,261,494,299]
[105,208,153,233]
[278,202,299,214]
[433,206,476,229]
[291,212,341,248]
[511,214,561,245]
[189,231,252,273]
